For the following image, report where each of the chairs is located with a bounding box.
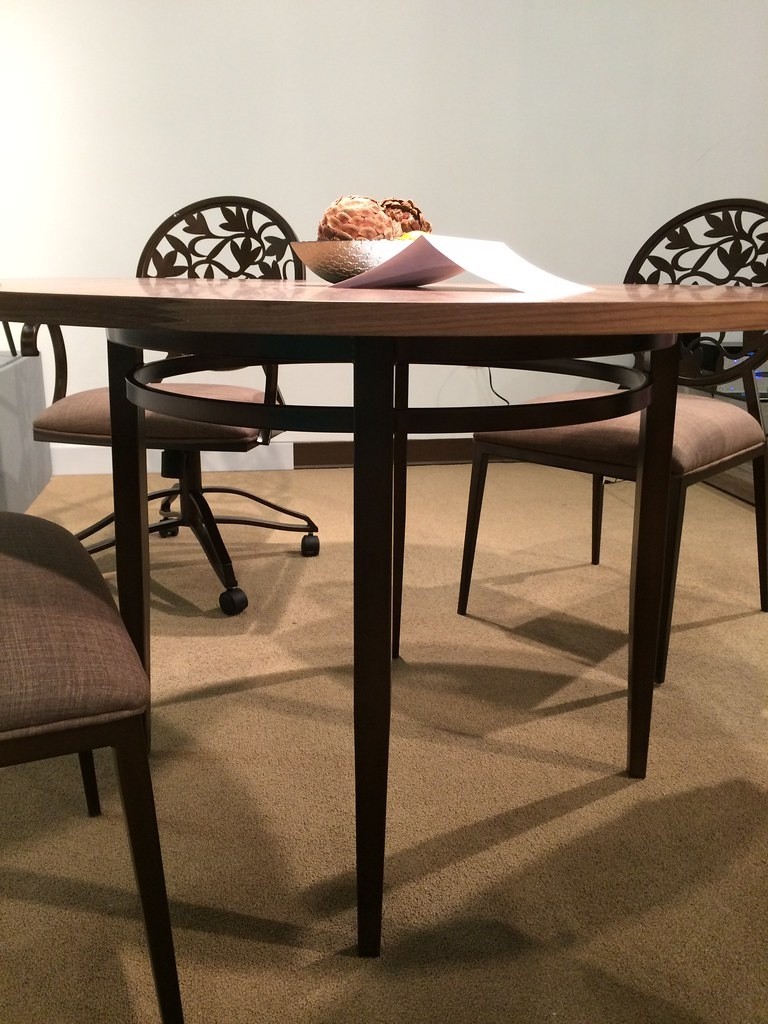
[1,511,184,1024]
[32,194,321,615]
[456,197,767,780]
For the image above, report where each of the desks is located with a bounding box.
[1,278,768,954]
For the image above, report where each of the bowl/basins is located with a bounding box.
[288,239,417,285]
